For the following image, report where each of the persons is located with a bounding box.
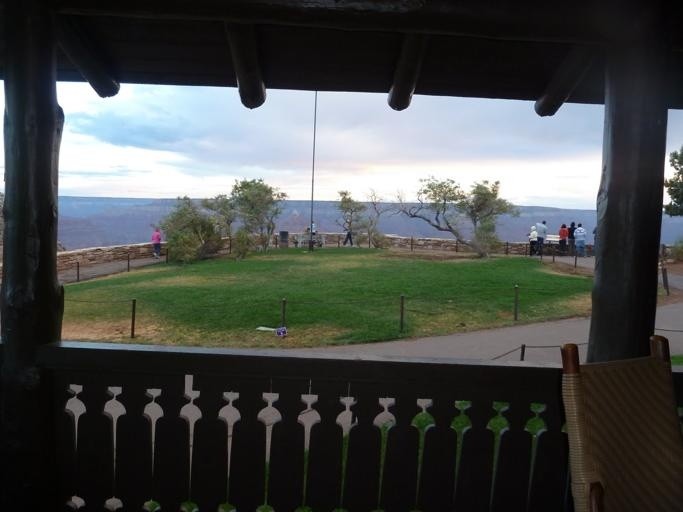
[591,226,597,257]
[151,227,161,259]
[567,221,578,257]
[342,219,355,248]
[573,222,587,257]
[308,220,318,245]
[526,225,538,257]
[535,220,548,256]
[558,223,569,257]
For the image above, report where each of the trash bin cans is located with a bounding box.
[279,231,288,248]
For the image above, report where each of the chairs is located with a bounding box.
[560,335,683,512]
